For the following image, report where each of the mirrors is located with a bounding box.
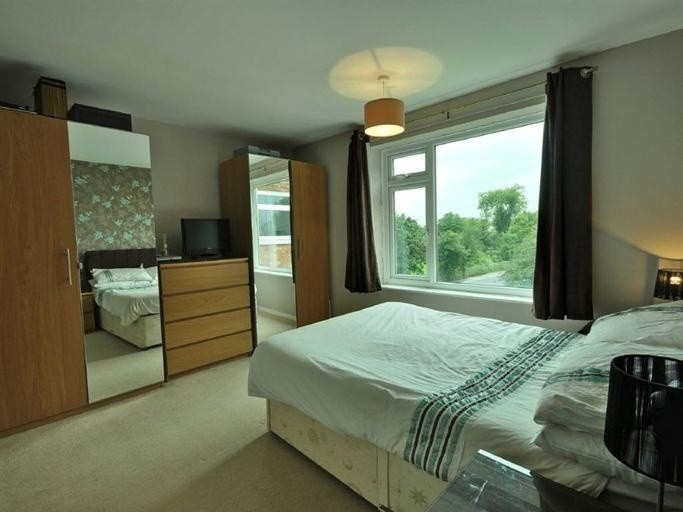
[68,120,167,405]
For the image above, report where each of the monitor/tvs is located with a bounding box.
[181,218,231,261]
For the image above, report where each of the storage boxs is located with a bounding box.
[68,102,132,132]
[33,76,68,120]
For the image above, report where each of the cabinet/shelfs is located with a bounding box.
[0,107,170,440]
[218,152,330,352]
[158,255,255,382]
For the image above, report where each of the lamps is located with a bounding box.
[363,74,405,138]
[652,267,682,300]
[248,153,298,347]
[603,354,682,512]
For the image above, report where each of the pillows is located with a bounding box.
[532,340,682,434]
[88,278,151,289]
[143,265,159,287]
[585,301,682,349]
[89,262,152,286]
[534,423,682,493]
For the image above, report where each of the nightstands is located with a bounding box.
[424,447,628,511]
[81,292,97,335]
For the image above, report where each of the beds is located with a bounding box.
[246,299,683,511]
[85,247,163,351]
